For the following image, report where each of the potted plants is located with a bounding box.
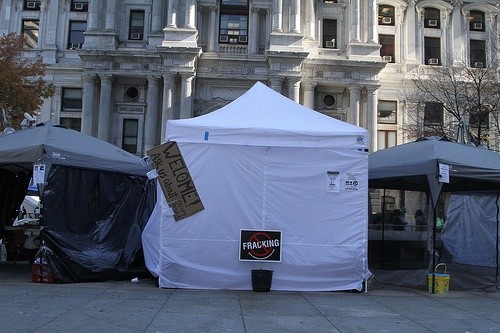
[251,268,275,292]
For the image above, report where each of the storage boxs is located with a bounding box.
[32,263,53,283]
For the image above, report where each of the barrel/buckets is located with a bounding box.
[428,264,450,294]
[251,269,274,292]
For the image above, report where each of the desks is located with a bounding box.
[5,227,39,265]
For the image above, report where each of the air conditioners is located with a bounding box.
[220,35,228,42]
[473,23,482,29]
[75,3,83,9]
[27,2,36,8]
[72,42,80,49]
[131,33,140,39]
[325,41,335,47]
[428,58,438,64]
[428,20,438,26]
[382,56,392,62]
[475,62,483,67]
[381,17,391,24]
[239,36,247,42]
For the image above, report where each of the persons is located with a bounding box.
[414,209,426,231]
[392,210,408,231]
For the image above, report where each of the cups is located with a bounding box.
[131,278,138,282]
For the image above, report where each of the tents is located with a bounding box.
[142,81,369,292]
[0,120,157,284]
[368,135,500,293]
